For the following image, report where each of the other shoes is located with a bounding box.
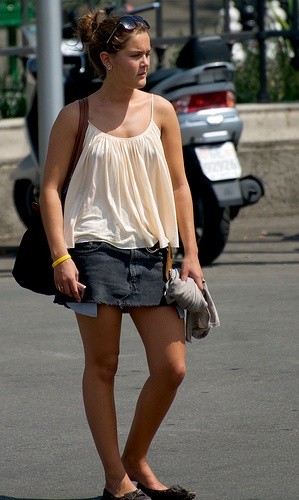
[136,481,197,500]
[102,488,153,500]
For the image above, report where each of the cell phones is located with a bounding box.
[68,281,86,299]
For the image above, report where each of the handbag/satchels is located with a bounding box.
[11,201,71,298]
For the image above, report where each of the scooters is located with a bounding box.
[9,2,265,268]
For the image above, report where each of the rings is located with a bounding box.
[202,279,206,283]
[57,285,64,292]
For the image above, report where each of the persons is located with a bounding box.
[38,8,204,500]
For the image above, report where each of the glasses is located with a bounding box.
[104,14,151,49]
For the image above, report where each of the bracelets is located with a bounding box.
[51,254,71,269]
